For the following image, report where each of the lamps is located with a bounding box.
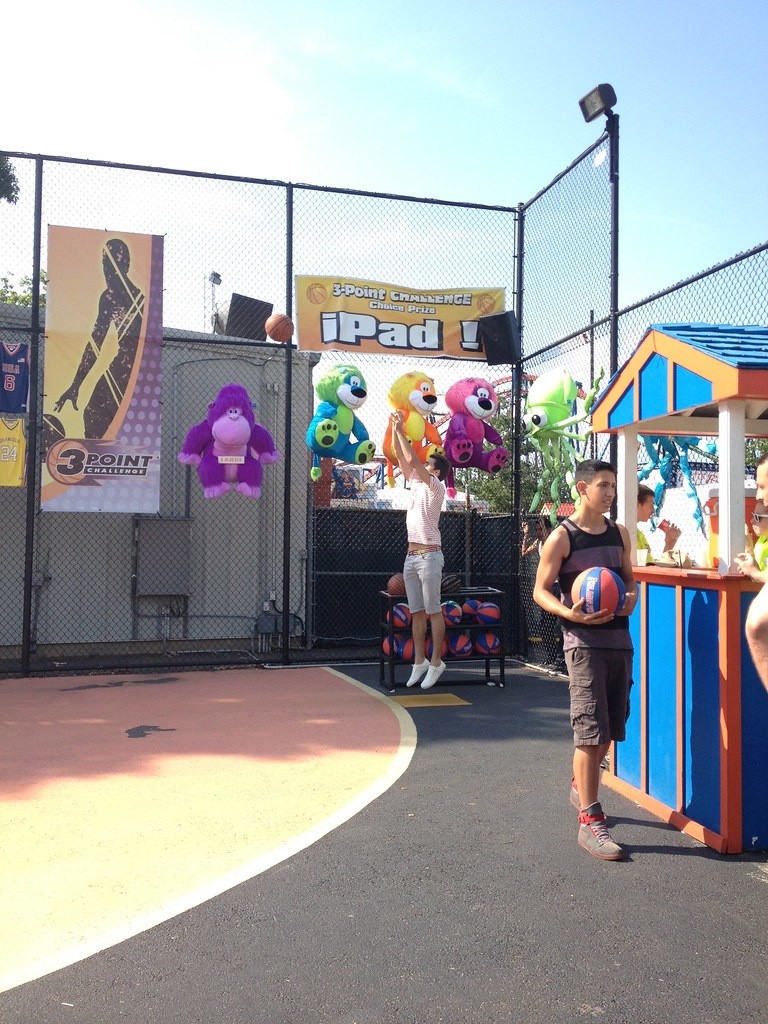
[208,271,222,285]
[577,82,617,123]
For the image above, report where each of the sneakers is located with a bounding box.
[577,802,624,860]
[570,776,608,827]
[406,658,430,687]
[420,660,446,690]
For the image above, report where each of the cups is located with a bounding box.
[637,548,648,567]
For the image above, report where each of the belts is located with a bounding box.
[407,546,441,555]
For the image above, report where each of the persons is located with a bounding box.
[521,516,567,674]
[533,459,639,860]
[387,409,450,690]
[637,483,682,566]
[733,452,768,696]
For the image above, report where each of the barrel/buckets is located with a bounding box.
[703,489,758,567]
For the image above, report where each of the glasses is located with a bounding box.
[751,512,768,522]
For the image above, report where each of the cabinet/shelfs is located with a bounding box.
[378,586,506,694]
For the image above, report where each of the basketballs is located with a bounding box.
[387,573,407,594]
[386,599,500,627]
[382,633,501,660]
[570,567,626,617]
[264,313,294,343]
[441,574,462,594]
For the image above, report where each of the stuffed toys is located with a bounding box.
[177,383,280,500]
[383,370,446,489]
[305,364,376,482]
[444,377,509,499]
[522,367,605,527]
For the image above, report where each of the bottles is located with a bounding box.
[650,515,683,541]
